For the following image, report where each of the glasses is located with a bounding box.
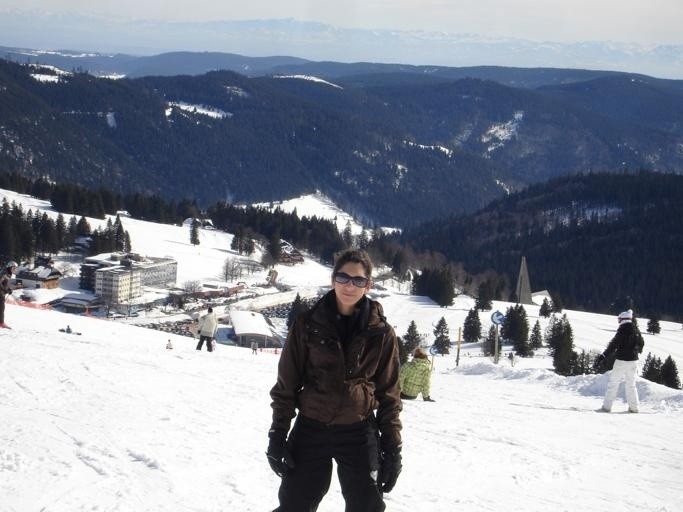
[332,269,370,289]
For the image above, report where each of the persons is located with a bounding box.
[265,247,403,512]
[398,346,438,403]
[591,309,644,413]
[0,261,18,329]
[193,306,218,352]
[251,339,258,356]
[210,336,217,352]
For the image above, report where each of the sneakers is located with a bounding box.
[602,406,638,414]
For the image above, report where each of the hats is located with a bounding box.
[617,309,634,320]
[414,346,428,359]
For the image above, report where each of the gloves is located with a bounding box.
[263,425,291,478]
[423,396,436,402]
[375,436,403,493]
